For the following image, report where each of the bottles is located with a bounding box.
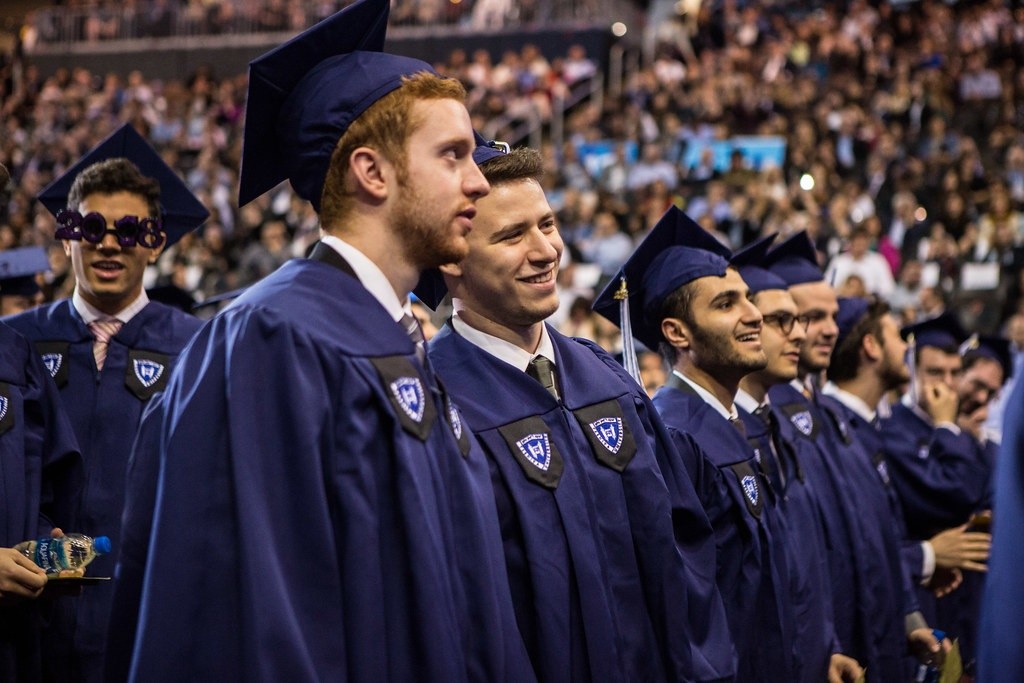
[907,629,947,683]
[18,533,112,575]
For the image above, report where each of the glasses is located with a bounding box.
[53,210,163,249]
[763,313,811,337]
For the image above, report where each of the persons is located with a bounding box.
[108,0,535,683]
[0,123,212,683]
[590,205,864,682]
[410,131,740,681]
[0,0,1024,683]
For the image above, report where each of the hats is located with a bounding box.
[836,294,867,329]
[0,246,58,299]
[413,128,510,311]
[961,330,1016,384]
[899,308,974,400]
[239,0,446,214]
[732,230,790,295]
[767,228,827,284]
[592,205,732,394]
[35,122,211,252]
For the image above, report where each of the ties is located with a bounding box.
[399,313,427,369]
[528,357,561,407]
[87,320,122,371]
[728,417,748,442]
